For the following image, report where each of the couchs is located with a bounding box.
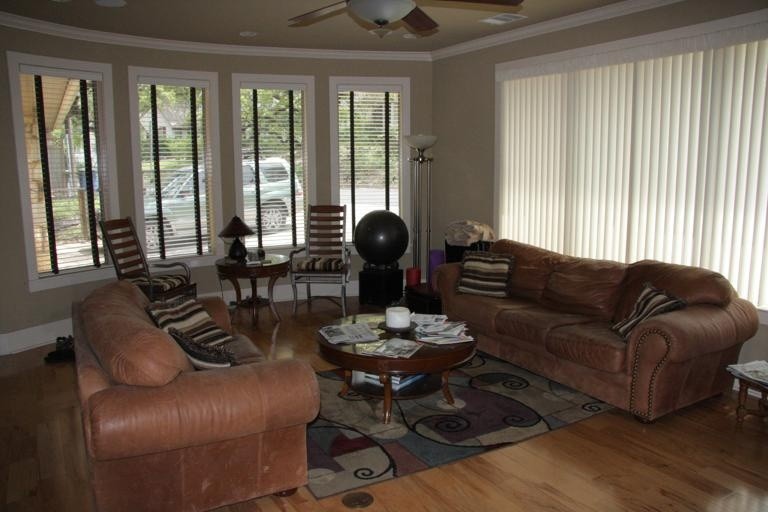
[68,279,322,512]
[432,238,761,428]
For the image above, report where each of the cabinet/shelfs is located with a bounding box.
[358,268,404,308]
[406,284,441,315]
[444,240,493,264]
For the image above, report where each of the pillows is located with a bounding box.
[456,249,515,300]
[144,296,217,334]
[167,326,238,371]
[609,279,688,344]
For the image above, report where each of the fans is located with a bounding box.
[287,0,523,40]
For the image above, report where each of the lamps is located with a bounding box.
[345,1,418,30]
[216,214,256,260]
[402,131,439,288]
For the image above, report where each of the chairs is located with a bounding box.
[95,216,199,302]
[286,204,353,317]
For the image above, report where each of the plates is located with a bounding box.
[378,320,419,335]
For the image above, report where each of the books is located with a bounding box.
[410,311,475,346]
[362,373,425,392]
[246,259,272,265]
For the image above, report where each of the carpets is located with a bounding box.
[293,350,617,501]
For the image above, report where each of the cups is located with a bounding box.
[406,267,421,287]
[429,249,444,284]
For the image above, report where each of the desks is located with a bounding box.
[214,253,291,329]
[729,370,768,425]
[317,312,480,426]
